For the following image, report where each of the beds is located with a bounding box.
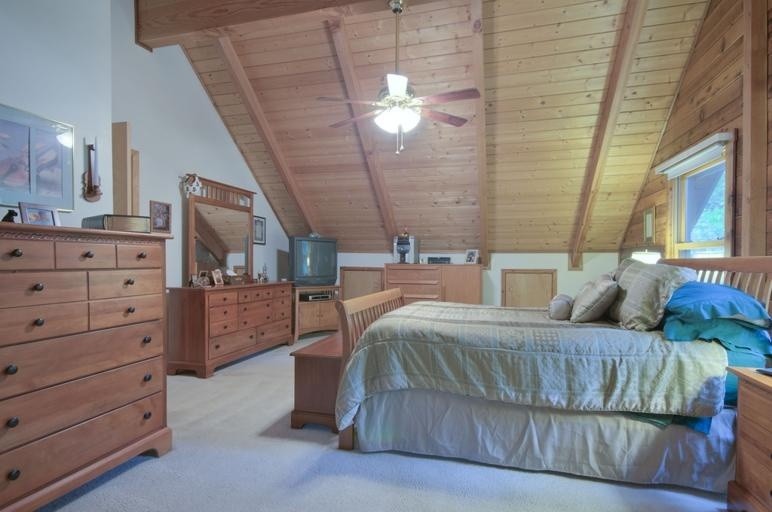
[334,256,772,495]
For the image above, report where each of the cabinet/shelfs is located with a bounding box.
[292,286,341,343]
[166,281,296,378]
[385,263,483,304]
[0,221,174,512]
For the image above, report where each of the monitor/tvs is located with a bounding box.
[287,234,338,287]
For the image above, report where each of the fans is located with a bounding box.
[317,0,480,128]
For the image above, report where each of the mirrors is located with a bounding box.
[185,174,258,279]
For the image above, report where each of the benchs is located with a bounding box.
[290,332,342,433]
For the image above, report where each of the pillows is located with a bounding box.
[548,294,573,320]
[606,257,697,331]
[660,314,772,359]
[570,274,619,323]
[665,280,772,330]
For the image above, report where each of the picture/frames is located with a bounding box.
[252,215,266,245]
[18,202,61,226]
[0,103,75,213]
[464,250,478,264]
[150,200,172,233]
[211,270,224,284]
[199,271,208,277]
[643,206,655,243]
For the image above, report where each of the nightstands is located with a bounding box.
[725,366,772,512]
